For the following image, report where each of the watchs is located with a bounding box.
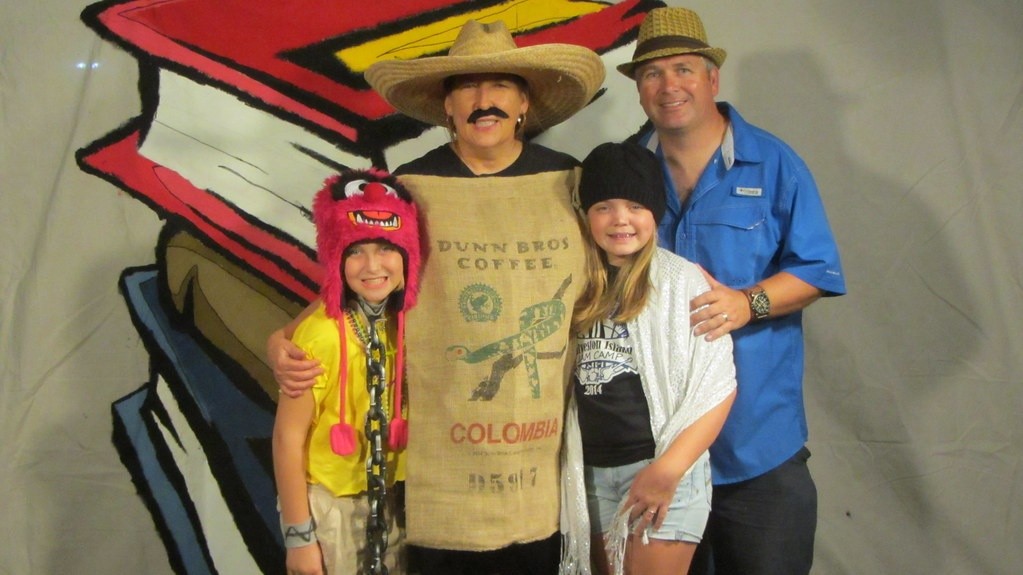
[742,285,771,324]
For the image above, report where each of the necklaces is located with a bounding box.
[343,305,408,420]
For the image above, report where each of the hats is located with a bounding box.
[364,18,606,133]
[579,141,668,225]
[616,6,728,79]
[312,165,422,455]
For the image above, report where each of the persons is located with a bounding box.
[265,23,606,575]
[558,142,738,575]
[272,170,420,575]
[617,7,846,574]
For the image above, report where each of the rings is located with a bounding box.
[722,313,728,321]
[647,509,656,514]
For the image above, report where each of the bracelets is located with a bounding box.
[283,521,317,548]
